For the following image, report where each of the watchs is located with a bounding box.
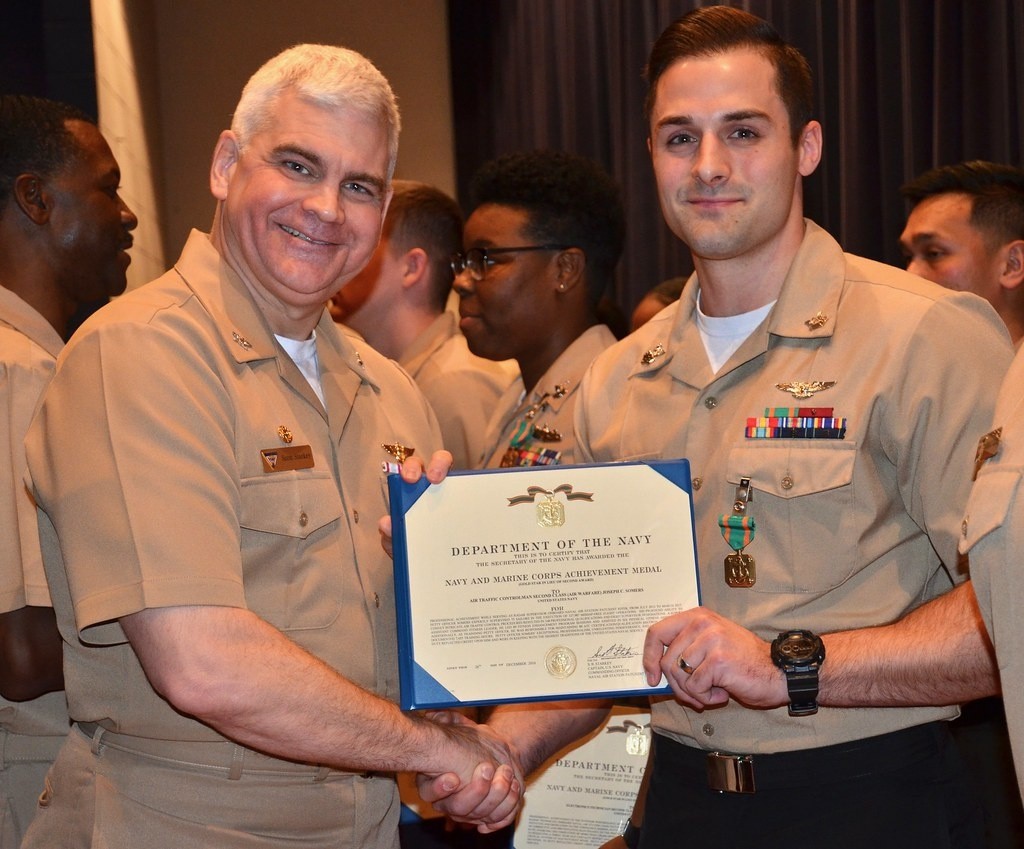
[770,629,826,716]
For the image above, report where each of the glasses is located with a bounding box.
[450,241,572,280]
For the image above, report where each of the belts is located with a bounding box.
[650,720,963,794]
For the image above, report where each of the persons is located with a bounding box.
[0,93,139,849]
[21,44,524,849]
[416,7,1024,849]
[328,149,617,472]
